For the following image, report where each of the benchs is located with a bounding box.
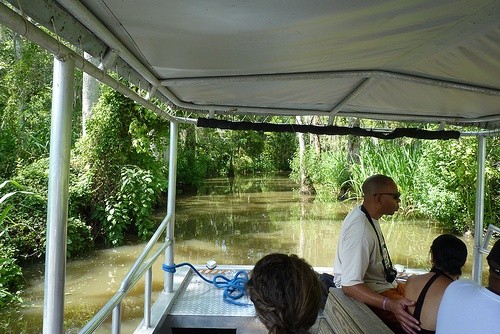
[318,287,393,334]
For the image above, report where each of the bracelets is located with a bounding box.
[382,297,388,310]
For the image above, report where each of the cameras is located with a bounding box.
[386,267,397,283]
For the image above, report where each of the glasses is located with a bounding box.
[373,192,401,200]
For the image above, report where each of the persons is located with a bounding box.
[333,174,422,334]
[243,252,327,334]
[399,234,467,334]
[435,238,500,334]
